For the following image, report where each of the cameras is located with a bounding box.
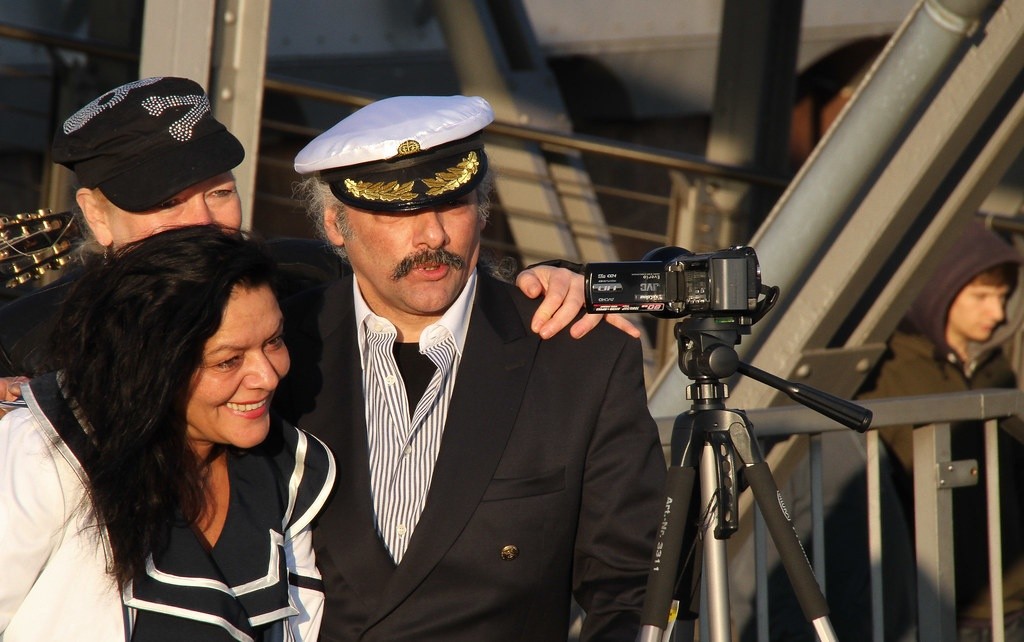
[585,246,760,318]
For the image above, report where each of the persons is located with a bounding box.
[0,224,336,642]
[0,97,668,642]
[853,222,1024,614]
[0,76,641,416]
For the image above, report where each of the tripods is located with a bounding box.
[637,317,873,642]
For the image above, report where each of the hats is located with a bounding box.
[51,77,244,213]
[294,95,493,215]
